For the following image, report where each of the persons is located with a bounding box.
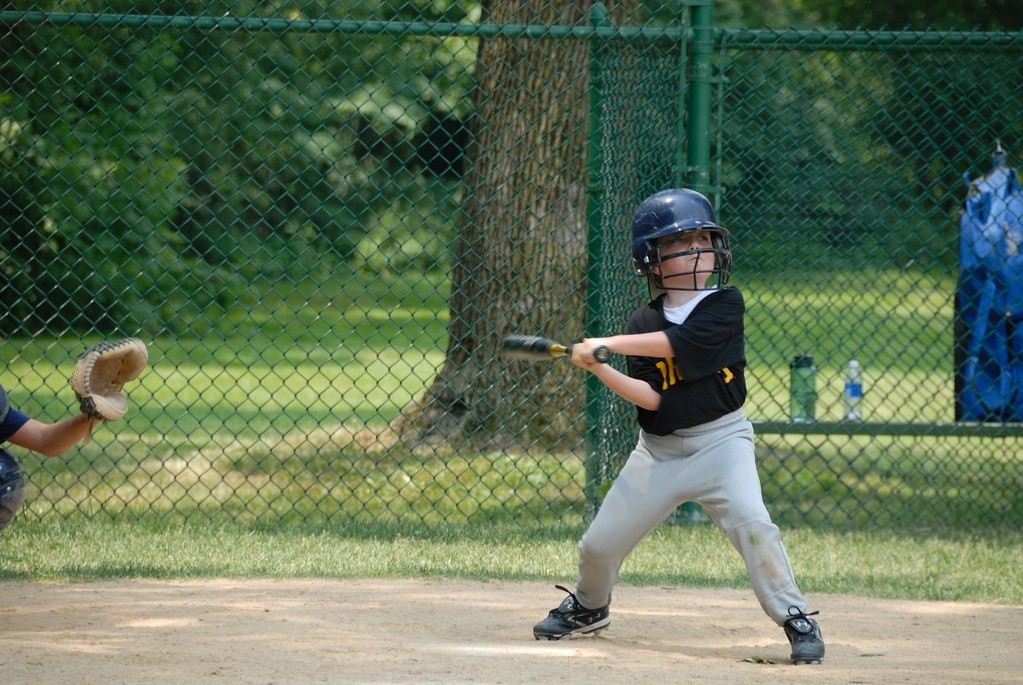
[533,189,826,665]
[0,337,148,528]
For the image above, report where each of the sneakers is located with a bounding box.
[533,585,611,640]
[782,606,825,664]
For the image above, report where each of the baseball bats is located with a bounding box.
[501,333,612,365]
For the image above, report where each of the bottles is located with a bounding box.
[790,354,816,425]
[843,359,863,423]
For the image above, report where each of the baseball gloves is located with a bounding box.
[69,337,149,423]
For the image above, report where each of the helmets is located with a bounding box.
[632,188,728,278]
